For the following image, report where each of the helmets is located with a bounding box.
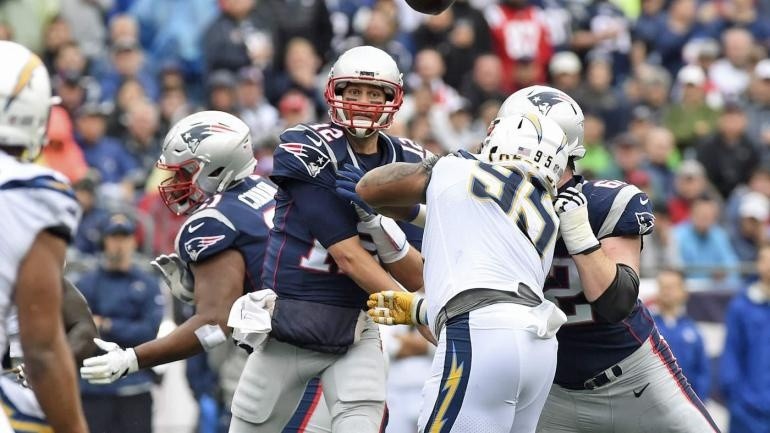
[498,86,586,162]
[2,35,53,161]
[479,109,571,186]
[326,44,403,132]
[156,110,259,216]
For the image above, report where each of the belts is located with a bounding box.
[560,364,621,391]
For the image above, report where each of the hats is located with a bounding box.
[103,213,135,235]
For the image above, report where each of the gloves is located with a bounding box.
[366,290,415,326]
[78,337,140,385]
[557,187,602,257]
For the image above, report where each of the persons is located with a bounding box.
[0,0,770,433]
